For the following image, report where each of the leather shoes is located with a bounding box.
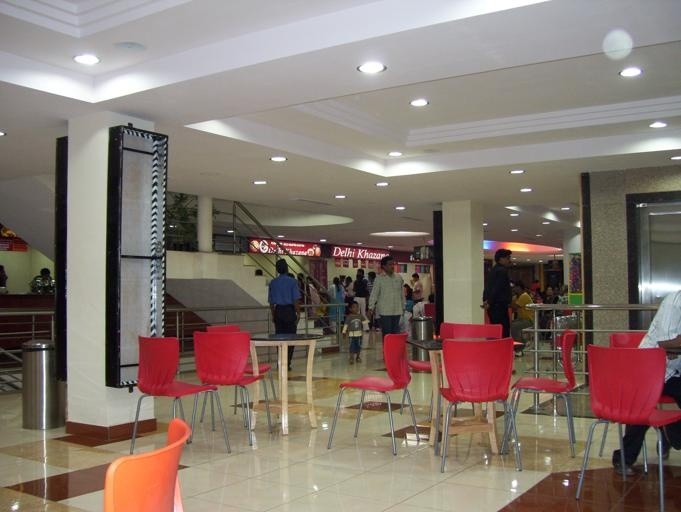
[615,463,636,475]
[653,426,670,459]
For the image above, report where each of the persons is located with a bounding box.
[368,255,405,361]
[289,267,377,336]
[611,288,681,476]
[342,300,370,365]
[30,267,54,294]
[267,258,302,373]
[401,273,435,317]
[479,249,516,375]
[254,269,263,275]
[508,277,567,357]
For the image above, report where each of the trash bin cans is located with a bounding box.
[21,338,57,430]
[410,315,433,373]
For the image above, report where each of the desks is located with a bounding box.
[247,331,324,435]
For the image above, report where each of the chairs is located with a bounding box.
[599,332,675,473]
[103,417,193,512]
[189,323,276,447]
[129,334,235,456]
[574,342,680,510]
[499,330,578,460]
[326,332,422,455]
[400,319,525,474]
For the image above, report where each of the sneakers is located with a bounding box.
[515,351,523,357]
